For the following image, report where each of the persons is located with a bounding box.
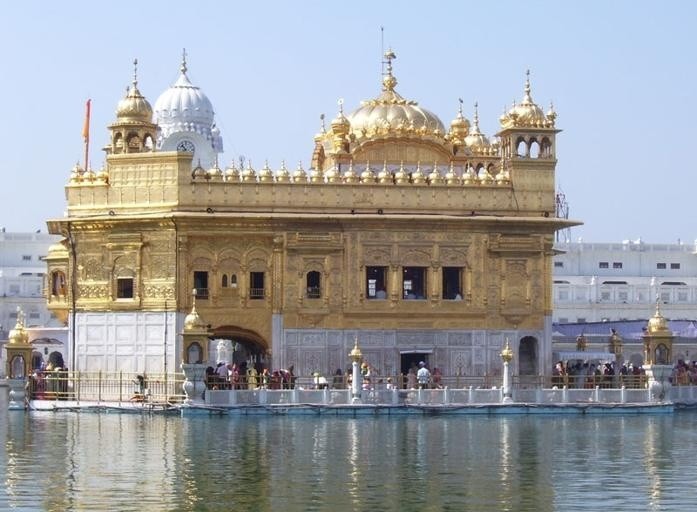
[552,359,697,389]
[206,360,443,389]
[32,362,68,400]
[374,288,461,299]
